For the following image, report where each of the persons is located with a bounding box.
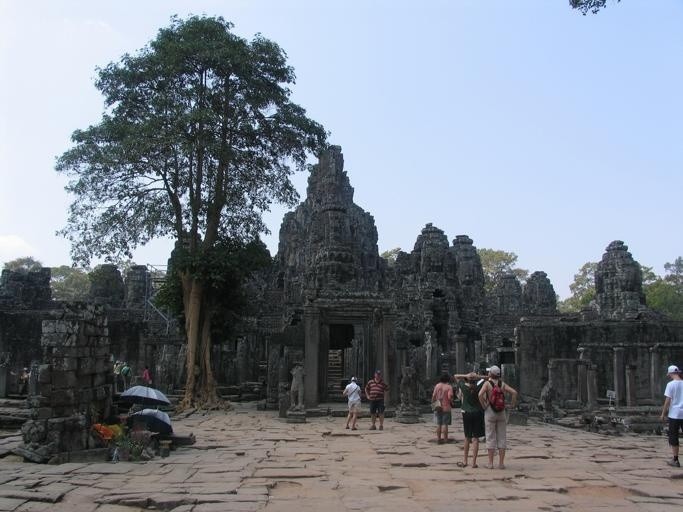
[660,363,682,468]
[363,368,388,432]
[430,374,454,445]
[113,361,152,387]
[477,366,517,470]
[343,376,361,431]
[455,377,484,442]
[453,373,488,469]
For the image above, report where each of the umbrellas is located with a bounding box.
[129,408,174,435]
[122,385,170,418]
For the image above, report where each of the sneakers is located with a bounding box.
[457,461,506,469]
[667,458,680,467]
[368,425,375,430]
[379,426,383,430]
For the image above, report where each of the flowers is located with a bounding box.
[92,420,148,463]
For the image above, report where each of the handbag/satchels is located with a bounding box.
[431,399,442,412]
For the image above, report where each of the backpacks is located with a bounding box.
[486,379,506,413]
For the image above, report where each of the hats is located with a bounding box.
[375,368,381,374]
[351,376,358,381]
[666,365,682,376]
